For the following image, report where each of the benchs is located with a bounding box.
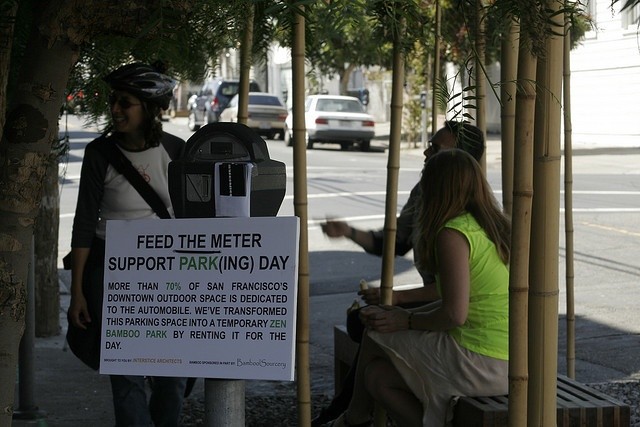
[334,322,640,427]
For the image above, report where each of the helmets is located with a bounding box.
[100,60,176,138]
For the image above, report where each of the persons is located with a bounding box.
[325,149,509,425]
[66,65,214,427]
[319,120,486,304]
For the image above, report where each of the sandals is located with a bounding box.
[322,410,372,427]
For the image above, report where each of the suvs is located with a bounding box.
[187,80,261,132]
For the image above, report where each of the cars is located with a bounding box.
[219,93,289,141]
[283,96,376,151]
